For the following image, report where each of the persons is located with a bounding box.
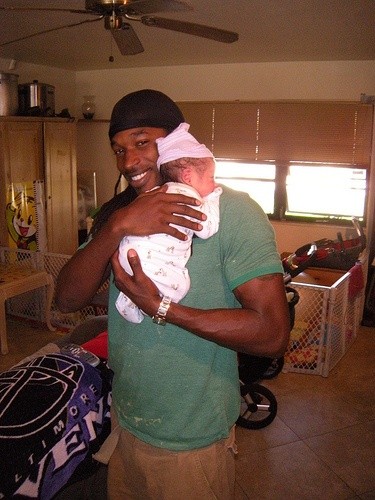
[115,122,223,323]
[53,88,289,500]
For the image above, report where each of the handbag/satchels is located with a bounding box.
[0,343,115,500]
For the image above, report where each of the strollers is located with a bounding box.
[235,243,317,430]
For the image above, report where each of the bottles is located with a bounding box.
[82,95,96,119]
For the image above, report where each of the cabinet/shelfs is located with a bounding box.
[0,115,79,256]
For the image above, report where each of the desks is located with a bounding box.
[0,262,54,355]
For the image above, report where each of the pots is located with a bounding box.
[0,72,20,117]
[19,79,56,116]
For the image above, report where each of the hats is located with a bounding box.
[155,123,215,172]
[108,88,184,142]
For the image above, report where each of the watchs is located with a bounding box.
[152,296,172,325]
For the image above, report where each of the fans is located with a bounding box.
[0,0,239,63]
